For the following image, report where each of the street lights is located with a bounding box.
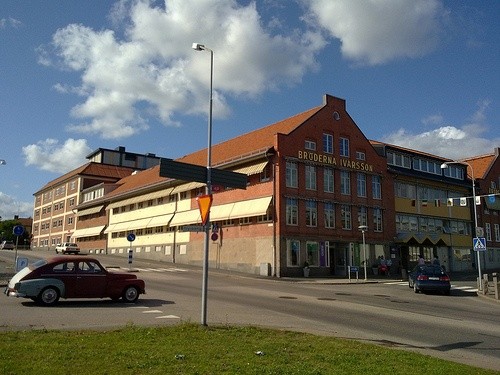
[192,43,214,327]
[441,161,482,291]
[358,225,368,280]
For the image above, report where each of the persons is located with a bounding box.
[418,255,425,264]
[431,256,440,265]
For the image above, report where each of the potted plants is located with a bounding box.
[372,265,378,276]
[303,261,310,277]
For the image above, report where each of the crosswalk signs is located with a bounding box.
[473,238,486,251]
[212,225,218,233]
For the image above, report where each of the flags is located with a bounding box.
[408,194,496,208]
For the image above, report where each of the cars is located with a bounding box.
[4,255,145,306]
[0,241,14,250]
[55,243,80,255]
[408,264,451,294]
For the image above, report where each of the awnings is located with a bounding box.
[71,159,272,239]
